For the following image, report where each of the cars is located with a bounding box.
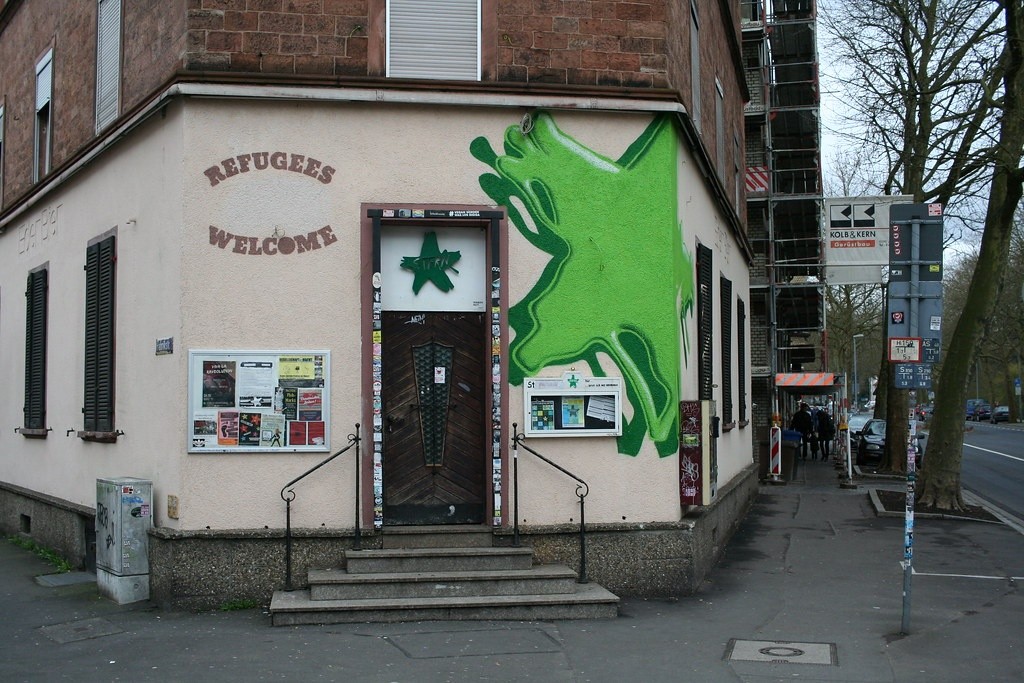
[990,406,1010,424]
[806,397,934,422]
[855,419,926,469]
[971,404,992,422]
[964,399,984,420]
[847,412,872,450]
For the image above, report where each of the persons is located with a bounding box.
[789,396,835,464]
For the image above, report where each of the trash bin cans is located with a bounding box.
[781,441,802,482]
[782,429,802,440]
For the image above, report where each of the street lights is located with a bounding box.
[853,333,863,413]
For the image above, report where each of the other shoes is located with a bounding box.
[795,454,829,462]
